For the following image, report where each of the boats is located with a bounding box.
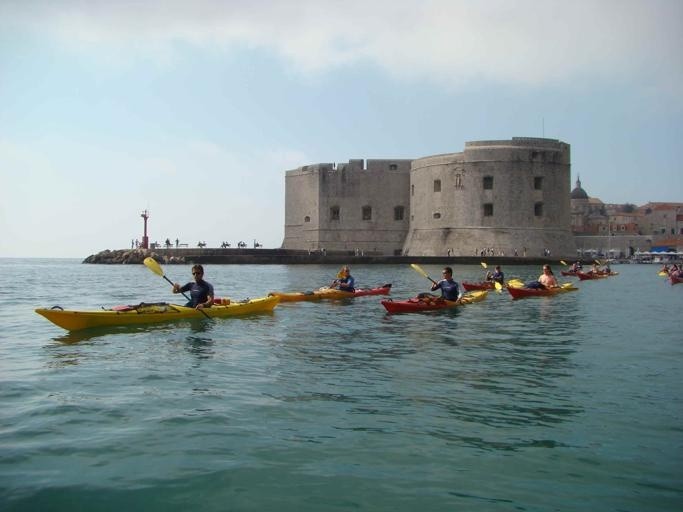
[561,271,619,279]
[656,270,683,284]
[462,279,521,291]
[35,296,280,329]
[381,290,489,313]
[505,282,575,298]
[269,284,393,302]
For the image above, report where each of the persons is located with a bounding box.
[447,247,552,257]
[538,263,558,288]
[568,259,611,277]
[172,265,215,311]
[430,267,460,302]
[661,262,683,281]
[491,265,504,285]
[131,238,260,249]
[333,264,355,292]
[308,247,366,257]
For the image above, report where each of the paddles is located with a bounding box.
[560,261,567,266]
[595,260,600,265]
[143,257,210,319]
[507,279,573,289]
[481,262,503,290]
[410,263,464,306]
[330,272,347,289]
